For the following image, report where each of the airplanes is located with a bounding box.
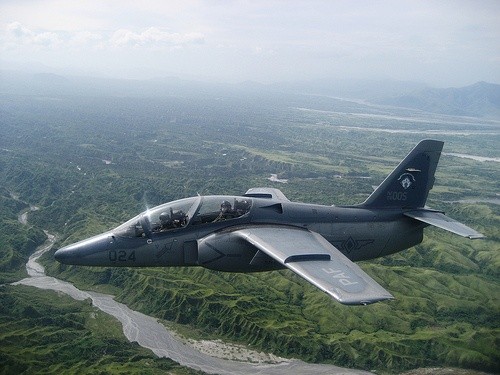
[54,138,486,305]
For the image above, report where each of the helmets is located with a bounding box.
[159,212,170,221]
[219,200,231,210]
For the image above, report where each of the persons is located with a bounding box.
[150,213,171,234]
[211,200,233,223]
